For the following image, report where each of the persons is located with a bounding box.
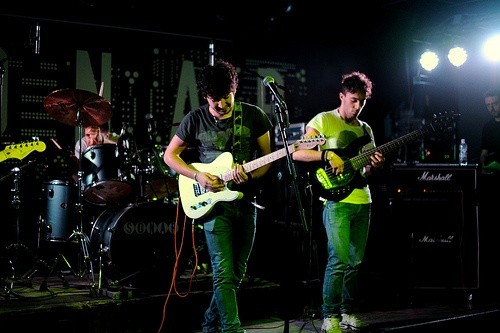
[73,121,120,181]
[480,90,500,157]
[163,61,272,333]
[291,71,385,333]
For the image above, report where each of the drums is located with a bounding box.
[119,125,179,200]
[36,179,87,247]
[89,201,194,291]
[74,145,139,205]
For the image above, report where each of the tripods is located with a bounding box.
[4,127,98,294]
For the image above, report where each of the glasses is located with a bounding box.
[486,102,498,108]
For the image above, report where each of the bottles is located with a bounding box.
[459,139,467,166]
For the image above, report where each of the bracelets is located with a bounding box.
[324,150,329,162]
[194,170,199,182]
[321,149,326,162]
[327,151,334,161]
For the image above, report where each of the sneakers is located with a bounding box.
[339,312,370,332]
[320,315,344,333]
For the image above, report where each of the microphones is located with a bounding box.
[263,76,286,110]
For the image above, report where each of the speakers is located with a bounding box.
[370,164,500,293]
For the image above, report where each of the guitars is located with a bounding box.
[0,141,47,165]
[178,136,327,221]
[312,109,462,202]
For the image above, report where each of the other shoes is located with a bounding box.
[201,323,221,333]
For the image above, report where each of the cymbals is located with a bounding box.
[43,88,112,126]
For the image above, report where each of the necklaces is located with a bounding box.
[212,112,223,123]
